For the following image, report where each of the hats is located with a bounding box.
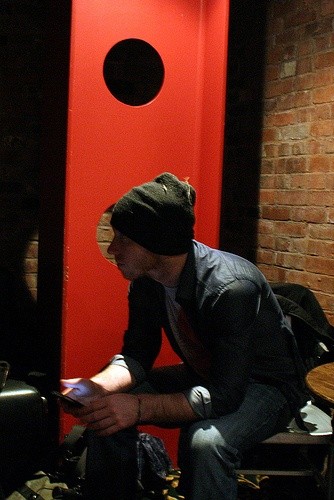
[111,171,197,255]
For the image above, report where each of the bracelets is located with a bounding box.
[136,395,144,427]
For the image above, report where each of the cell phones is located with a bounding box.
[53,391,84,409]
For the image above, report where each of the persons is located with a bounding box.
[51,172,311,500]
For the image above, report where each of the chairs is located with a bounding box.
[235,283,334,500]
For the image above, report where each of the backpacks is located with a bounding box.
[271,281,334,367]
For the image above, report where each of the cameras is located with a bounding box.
[52,475,86,500]
